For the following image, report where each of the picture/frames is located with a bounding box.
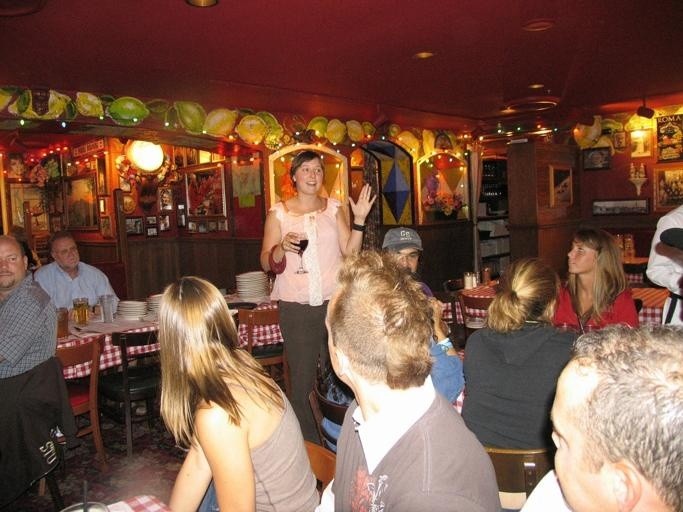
[548,163,573,209]
[122,214,144,234]
[653,168,683,213]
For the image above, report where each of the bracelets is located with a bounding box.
[351,223,367,232]
[437,338,454,351]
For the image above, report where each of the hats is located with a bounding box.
[383,228,424,252]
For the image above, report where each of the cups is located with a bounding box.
[463,272,473,290]
[56,307,68,341]
[293,233,309,275]
[99,295,114,324]
[73,297,89,327]
[472,273,477,288]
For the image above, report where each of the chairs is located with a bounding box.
[308,376,350,491]
[479,446,558,512]
[95,329,163,454]
[233,309,292,403]
[458,291,494,352]
[51,337,103,454]
[300,440,337,510]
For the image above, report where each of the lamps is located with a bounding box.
[124,137,168,174]
[505,84,558,112]
[186,0,218,8]
[636,97,656,121]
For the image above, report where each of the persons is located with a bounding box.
[6,149,27,176]
[259,151,377,441]
[647,199,683,325]
[383,227,432,297]
[462,259,585,451]
[159,276,320,512]
[160,190,173,209]
[0,235,57,383]
[590,152,605,167]
[324,252,504,512]
[518,324,683,511]
[35,231,124,314]
[555,224,644,329]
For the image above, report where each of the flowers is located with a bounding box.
[422,191,463,209]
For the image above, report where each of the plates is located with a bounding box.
[147,296,163,311]
[236,272,269,301]
[117,301,148,320]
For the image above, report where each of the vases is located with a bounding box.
[433,208,457,222]
